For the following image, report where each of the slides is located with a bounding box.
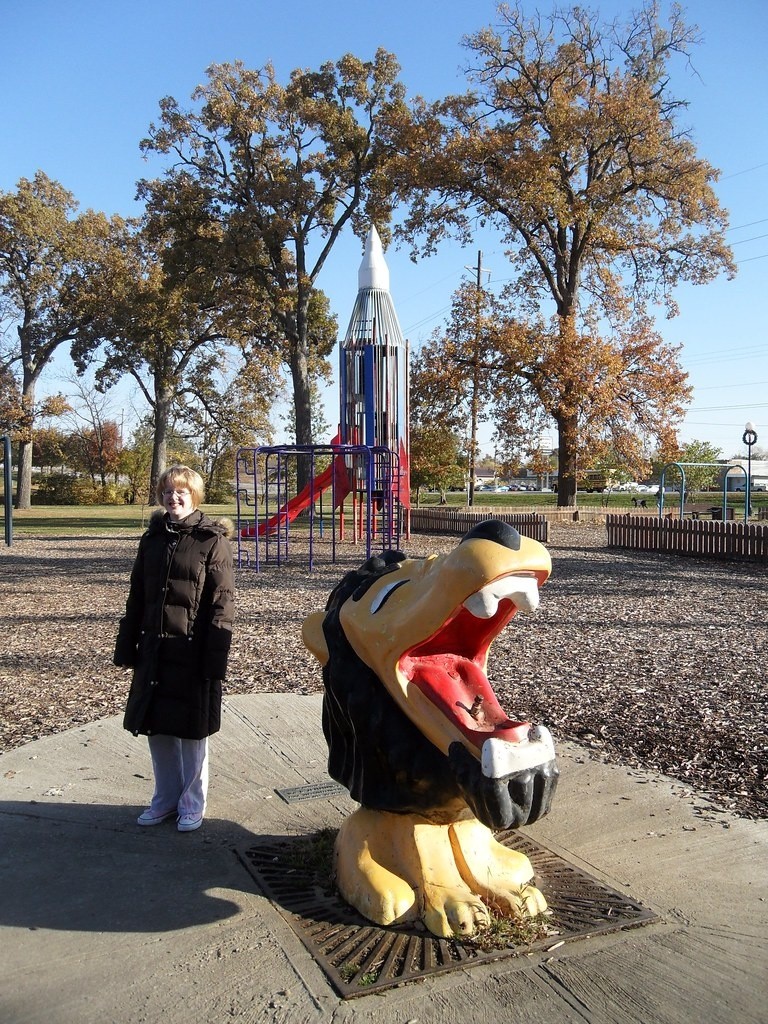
[241,433,344,540]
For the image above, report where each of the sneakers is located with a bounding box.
[176,806,205,831]
[137,808,176,825]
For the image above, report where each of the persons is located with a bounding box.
[654,487,665,508]
[112,465,235,832]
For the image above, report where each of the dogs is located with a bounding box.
[300,520,562,942]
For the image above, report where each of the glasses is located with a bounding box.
[162,489,191,498]
